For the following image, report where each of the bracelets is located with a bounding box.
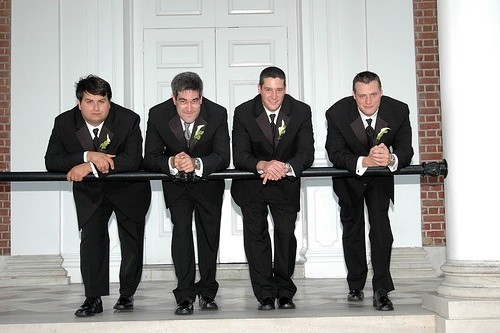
[391,156,395,166]
[285,164,292,172]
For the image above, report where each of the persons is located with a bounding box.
[144,72,231,315]
[230,66,315,310]
[324,71,414,311]
[45,76,152,316]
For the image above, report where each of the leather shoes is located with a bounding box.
[199,297,217,310]
[75,296,103,316]
[373,289,394,311]
[113,295,134,310]
[258,298,275,310]
[348,289,364,301]
[175,300,193,314]
[277,297,295,309]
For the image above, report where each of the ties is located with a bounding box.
[269,114,275,142]
[184,123,190,149]
[93,128,99,150]
[365,119,375,149]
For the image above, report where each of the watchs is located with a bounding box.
[195,159,200,170]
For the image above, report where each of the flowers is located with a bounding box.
[277,118,286,141]
[100,133,111,150]
[376,127,392,141]
[193,125,205,144]
